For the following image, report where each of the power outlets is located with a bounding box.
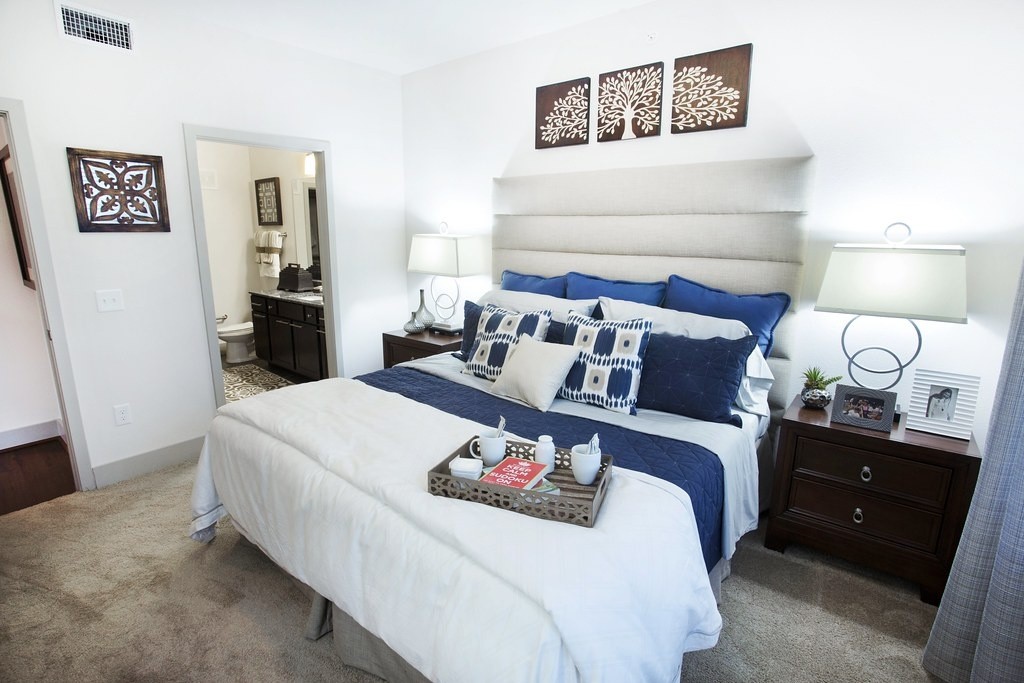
[115,404,132,425]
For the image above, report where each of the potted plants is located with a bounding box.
[801,366,842,411]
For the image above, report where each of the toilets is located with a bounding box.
[218,321,254,364]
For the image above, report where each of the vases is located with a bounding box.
[416,290,434,329]
[404,312,426,335]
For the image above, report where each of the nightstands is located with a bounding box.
[382,330,461,372]
[764,389,983,607]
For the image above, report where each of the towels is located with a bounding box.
[254,230,282,278]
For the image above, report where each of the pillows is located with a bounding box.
[662,276,789,356]
[499,270,566,298]
[640,334,760,428]
[556,309,652,416]
[599,294,772,419]
[459,304,552,380]
[489,334,585,413]
[452,299,566,361]
[564,272,669,320]
[476,285,599,325]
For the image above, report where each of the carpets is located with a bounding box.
[224,364,296,405]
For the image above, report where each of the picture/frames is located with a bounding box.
[904,369,984,441]
[66,146,173,233]
[832,384,898,433]
[254,176,283,227]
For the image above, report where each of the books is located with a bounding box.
[477,455,560,507]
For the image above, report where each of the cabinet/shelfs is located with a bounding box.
[250,295,329,380]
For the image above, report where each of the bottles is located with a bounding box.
[404,312,425,334]
[535,435,556,473]
[416,289,434,330]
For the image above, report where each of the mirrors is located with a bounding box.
[303,181,320,267]
[0,143,36,290]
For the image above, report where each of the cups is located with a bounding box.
[571,444,601,485]
[470,430,506,466]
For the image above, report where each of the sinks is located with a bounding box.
[296,294,323,302]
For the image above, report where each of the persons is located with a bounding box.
[846,396,884,421]
[927,388,953,421]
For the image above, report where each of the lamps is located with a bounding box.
[407,219,487,334]
[813,223,968,422]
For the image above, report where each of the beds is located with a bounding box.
[187,151,821,683]
